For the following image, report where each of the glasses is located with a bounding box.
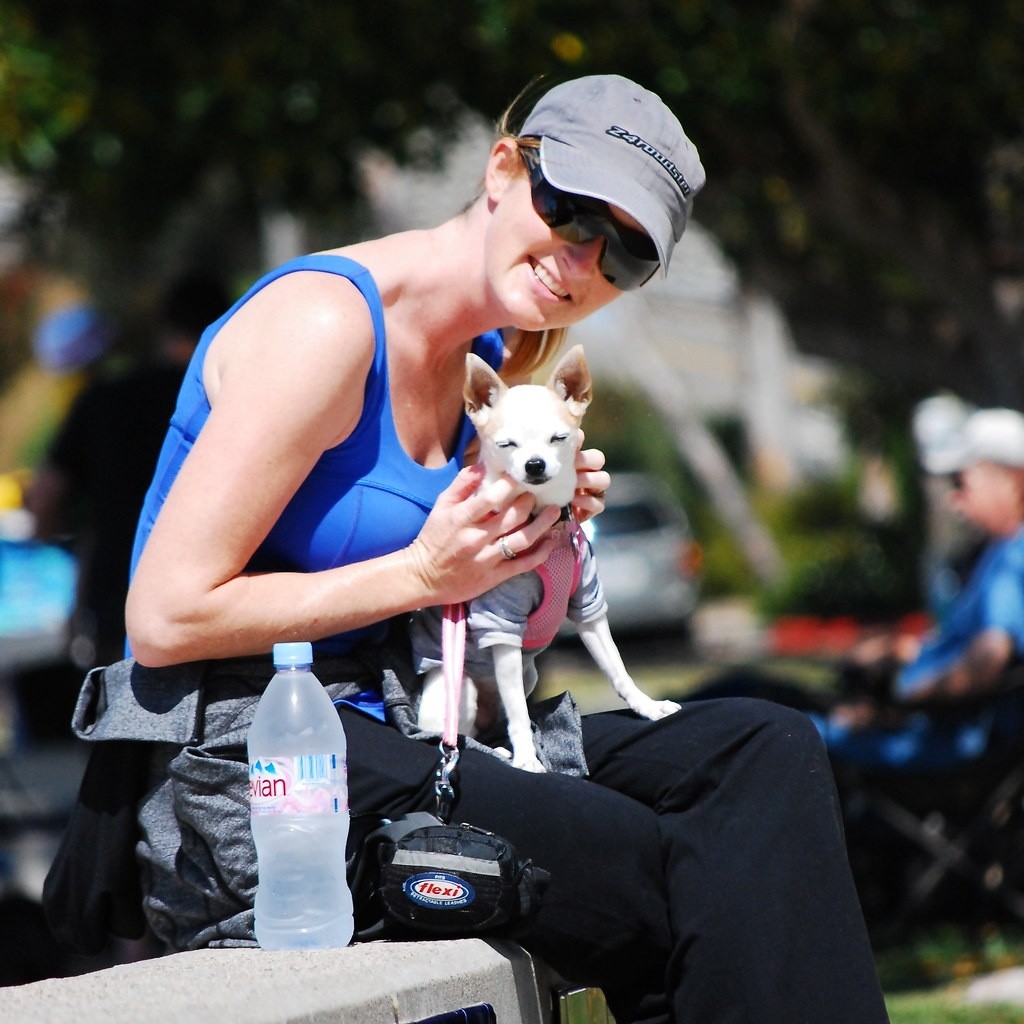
[520,143,660,292]
[951,467,997,489]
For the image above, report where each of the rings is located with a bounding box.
[498,535,517,560]
[584,488,605,498]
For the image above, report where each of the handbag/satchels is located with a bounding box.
[346,810,552,940]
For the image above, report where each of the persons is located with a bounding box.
[692,410,1024,812]
[122,71,894,1021]
[29,264,233,716]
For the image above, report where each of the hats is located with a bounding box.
[926,407,1024,474]
[518,74,708,282]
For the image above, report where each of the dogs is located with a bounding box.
[407,345,683,774]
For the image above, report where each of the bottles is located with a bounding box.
[246,641,355,953]
